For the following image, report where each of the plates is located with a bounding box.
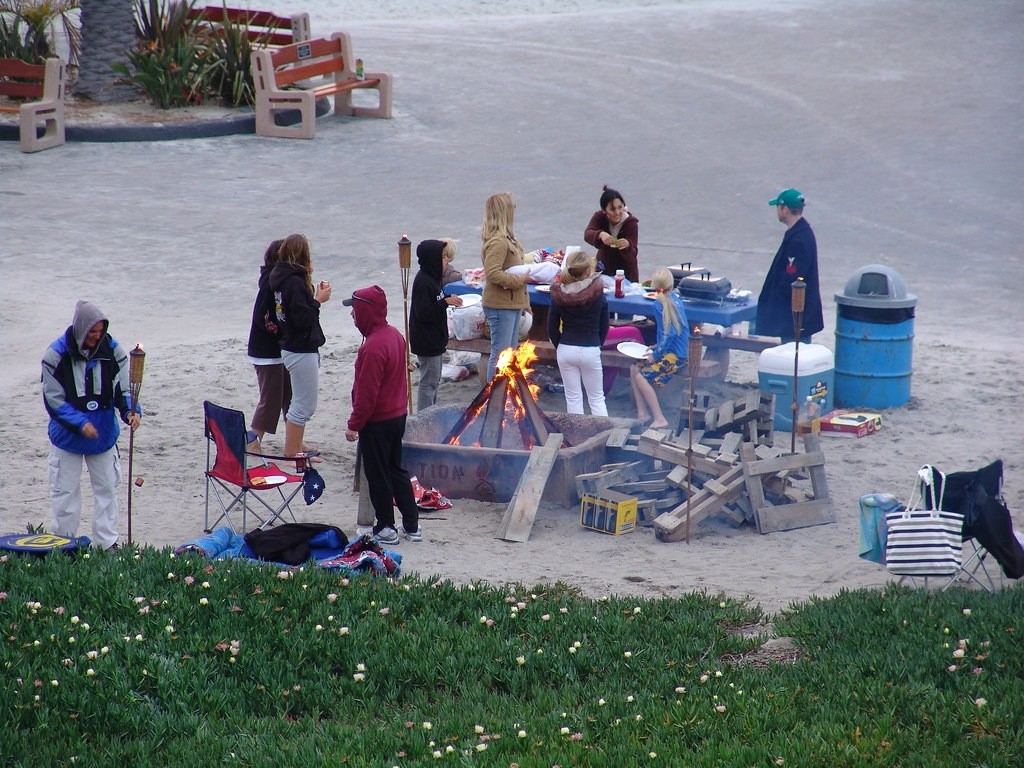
[264,476,287,484]
[617,341,653,360]
[535,285,551,293]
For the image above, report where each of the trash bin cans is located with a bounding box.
[833,264,919,409]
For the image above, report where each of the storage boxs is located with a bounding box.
[579,488,638,536]
[757,341,835,432]
[821,409,882,439]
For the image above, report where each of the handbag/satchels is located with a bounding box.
[886,464,964,576]
[446,304,533,341]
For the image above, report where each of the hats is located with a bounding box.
[768,189,805,208]
[343,299,353,306]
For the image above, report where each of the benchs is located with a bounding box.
[0,57,66,153]
[445,318,782,407]
[249,32,393,140]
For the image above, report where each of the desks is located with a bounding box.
[443,278,759,380]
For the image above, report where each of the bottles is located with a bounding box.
[614,269,625,298]
[643,292,659,300]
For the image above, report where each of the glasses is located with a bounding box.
[352,291,373,304]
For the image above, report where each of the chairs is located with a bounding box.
[203,400,326,541]
[860,458,1009,615]
[131,4,310,84]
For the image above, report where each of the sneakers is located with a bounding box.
[400,524,421,540]
[356,525,400,544]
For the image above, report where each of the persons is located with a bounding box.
[584,184,639,284]
[245,239,293,456]
[755,185,824,346]
[480,190,542,385]
[39,299,142,553]
[409,237,463,413]
[627,265,690,429]
[343,284,424,546]
[271,236,333,469]
[545,249,609,416]
